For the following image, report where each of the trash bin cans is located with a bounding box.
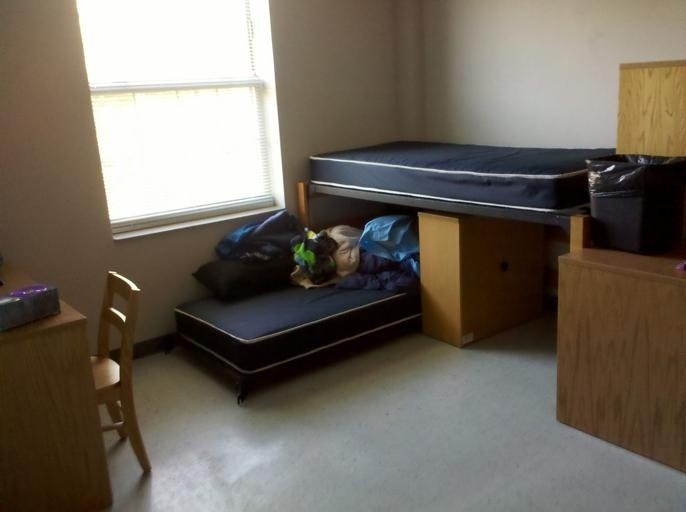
[585,154,686,254]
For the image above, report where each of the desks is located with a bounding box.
[0,269,112,512]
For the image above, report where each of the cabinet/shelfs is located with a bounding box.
[555,247,686,472]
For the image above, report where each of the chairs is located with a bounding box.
[90,271,152,474]
